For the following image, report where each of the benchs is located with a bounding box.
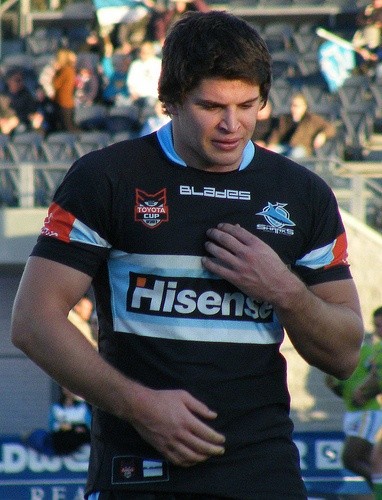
[0,0,382,207]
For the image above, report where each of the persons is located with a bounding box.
[0,1,382,499]
